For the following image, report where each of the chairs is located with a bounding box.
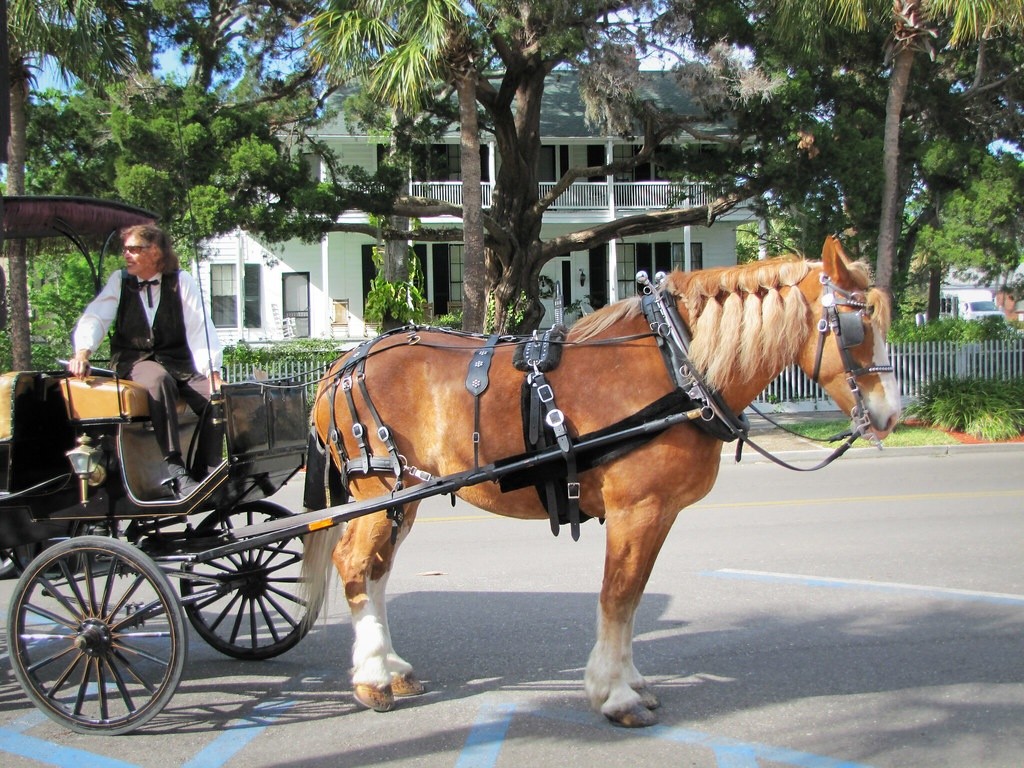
[330,298,351,339]
[271,304,298,341]
[362,298,379,338]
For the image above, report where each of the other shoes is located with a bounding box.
[173,472,200,496]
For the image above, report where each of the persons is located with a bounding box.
[70,225,227,501]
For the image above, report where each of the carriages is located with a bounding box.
[1,193,903,740]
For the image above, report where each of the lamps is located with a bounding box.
[580,270,586,285]
[66,431,105,503]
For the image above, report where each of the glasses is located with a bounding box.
[122,246,151,254]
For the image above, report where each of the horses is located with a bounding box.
[298,237,902,728]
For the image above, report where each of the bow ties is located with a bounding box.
[136,279,160,308]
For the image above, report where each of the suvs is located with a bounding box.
[960,300,1004,322]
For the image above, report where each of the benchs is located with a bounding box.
[60,375,193,502]
